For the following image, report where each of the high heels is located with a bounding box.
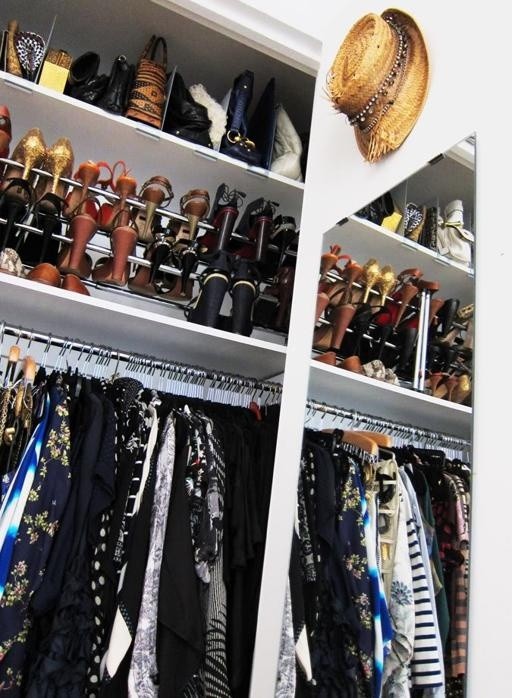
[427,344,442,373]
[399,279,444,328]
[374,268,423,329]
[94,160,138,233]
[315,278,335,325]
[350,258,380,305]
[228,258,262,337]
[54,196,102,281]
[31,137,75,216]
[158,237,199,296]
[320,244,351,281]
[0,104,13,174]
[0,127,46,202]
[92,208,140,287]
[328,302,374,357]
[425,368,444,396]
[327,259,363,306]
[429,297,460,339]
[0,178,33,253]
[274,266,296,328]
[363,305,392,361]
[397,316,418,366]
[17,192,69,264]
[183,250,236,328]
[369,263,397,314]
[435,372,459,401]
[161,188,211,242]
[227,198,280,264]
[127,227,177,298]
[129,175,175,242]
[265,213,295,272]
[199,183,246,253]
[439,303,475,351]
[62,159,113,220]
[313,287,356,350]
[442,348,455,372]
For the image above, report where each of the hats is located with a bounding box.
[325,7,431,164]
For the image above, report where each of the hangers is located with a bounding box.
[307,402,470,463]
[0,322,283,410]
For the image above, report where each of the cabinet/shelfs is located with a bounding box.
[1,0,320,380]
[271,145,473,433]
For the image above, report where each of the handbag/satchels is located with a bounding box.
[125,33,171,132]
[221,67,258,165]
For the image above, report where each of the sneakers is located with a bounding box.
[314,350,337,367]
[338,356,364,374]
[23,262,91,298]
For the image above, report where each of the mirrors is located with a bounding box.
[273,131,476,697]
